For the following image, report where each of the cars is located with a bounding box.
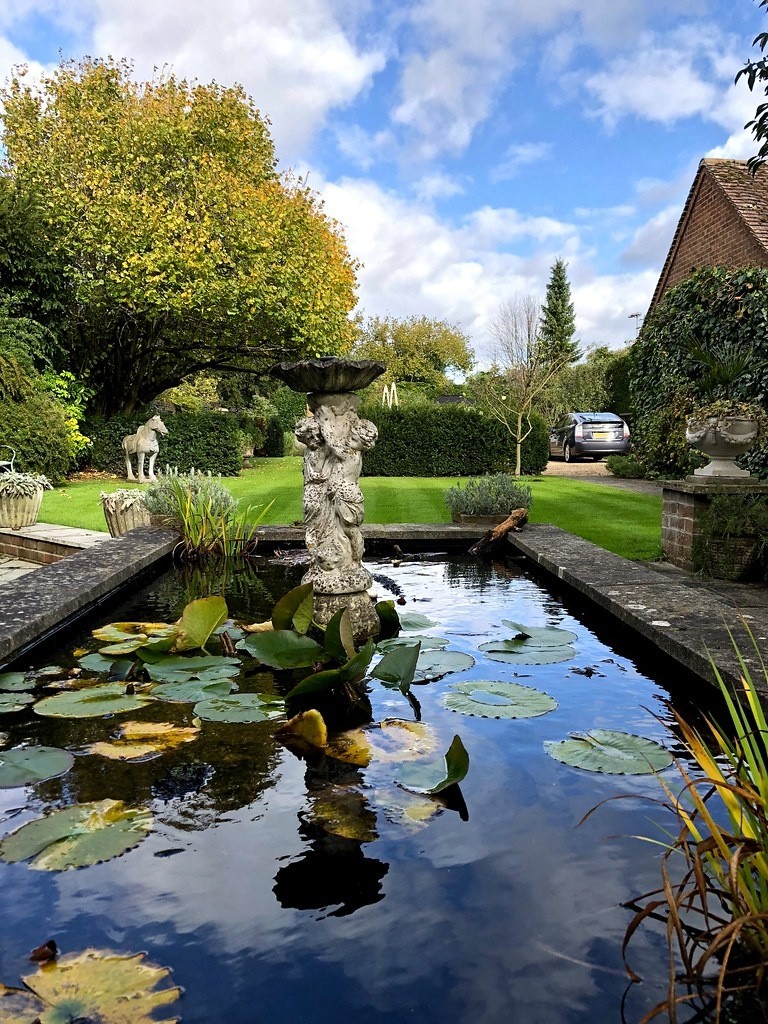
[547,412,631,463]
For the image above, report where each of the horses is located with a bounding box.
[121,415,169,486]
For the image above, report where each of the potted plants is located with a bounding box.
[0,449,54,528]
[677,336,768,476]
[695,482,768,581]
[99,463,236,538]
[443,470,533,525]
